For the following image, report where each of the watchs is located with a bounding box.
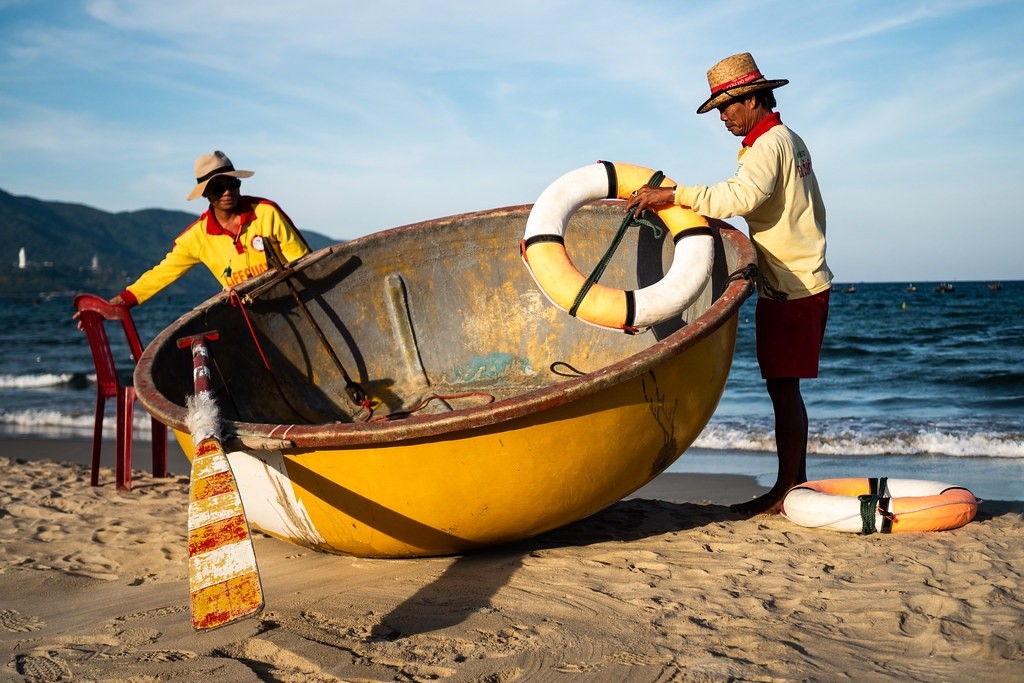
[672,186,676,205]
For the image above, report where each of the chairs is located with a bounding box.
[72,292,168,489]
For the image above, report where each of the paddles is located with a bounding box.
[177,329,266,632]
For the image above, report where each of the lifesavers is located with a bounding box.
[780,477,978,536]
[520,159,715,336]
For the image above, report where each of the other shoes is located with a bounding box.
[730,486,791,514]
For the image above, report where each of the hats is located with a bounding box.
[188,151,255,201]
[697,52,789,113]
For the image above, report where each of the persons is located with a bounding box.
[624,51,834,516]
[72,150,312,332]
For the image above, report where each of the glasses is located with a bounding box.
[208,178,242,195]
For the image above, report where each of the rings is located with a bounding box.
[632,190,637,197]
